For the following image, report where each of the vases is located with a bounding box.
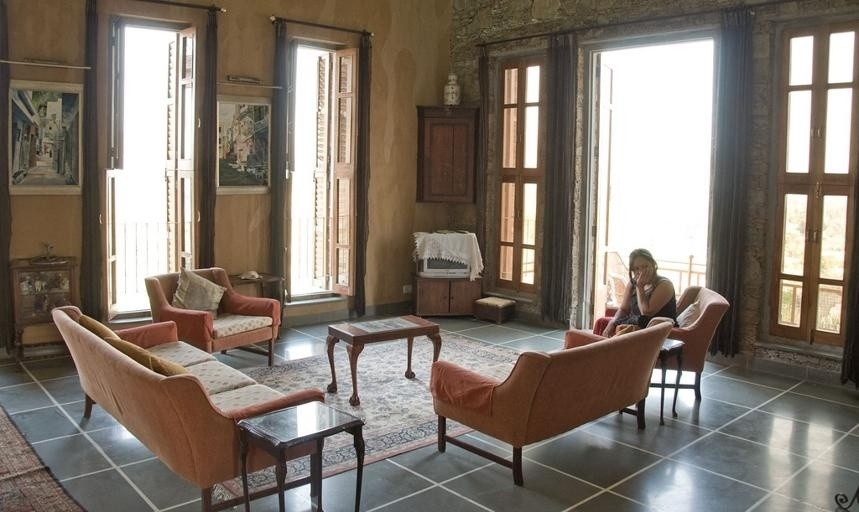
[444,73,463,106]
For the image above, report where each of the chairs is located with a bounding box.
[605,287,732,401]
[143,266,283,366]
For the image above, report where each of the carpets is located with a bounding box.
[224,334,518,496]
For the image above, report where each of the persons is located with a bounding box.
[600,248,677,338]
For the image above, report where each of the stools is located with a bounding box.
[474,296,516,325]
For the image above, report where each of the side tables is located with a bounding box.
[657,338,686,427]
[234,401,365,512]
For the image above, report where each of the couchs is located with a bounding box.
[429,313,677,488]
[50,305,326,512]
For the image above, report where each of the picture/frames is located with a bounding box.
[214,94,274,195]
[8,78,85,198]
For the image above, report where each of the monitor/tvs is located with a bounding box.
[419,233,475,278]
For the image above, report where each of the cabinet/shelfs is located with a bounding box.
[413,277,483,319]
[414,104,481,205]
[12,256,79,373]
